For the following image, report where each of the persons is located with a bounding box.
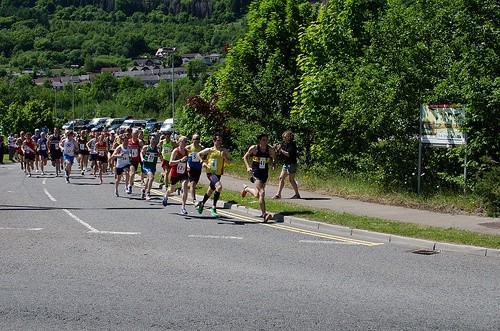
[271,130,301,198]
[241,133,276,222]
[0,126,232,218]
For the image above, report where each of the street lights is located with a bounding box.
[72,81,80,133]
[78,89,85,130]
[52,86,57,126]
[155,47,176,140]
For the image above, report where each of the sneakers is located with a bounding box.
[210,207,218,217]
[241,185,248,197]
[264,213,271,222]
[198,203,204,214]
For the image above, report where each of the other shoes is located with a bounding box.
[141,180,145,186]
[128,186,132,194]
[146,194,151,200]
[291,195,300,199]
[141,189,145,199]
[162,195,168,206]
[124,188,130,193]
[192,199,198,204]
[65,178,70,183]
[25,165,96,177]
[165,185,168,191]
[272,194,281,199]
[181,207,187,214]
[115,191,119,197]
[99,180,103,184]
[160,173,164,183]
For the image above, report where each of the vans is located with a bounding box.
[62,116,178,135]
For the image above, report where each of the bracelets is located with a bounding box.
[179,159,181,162]
[200,160,203,164]
